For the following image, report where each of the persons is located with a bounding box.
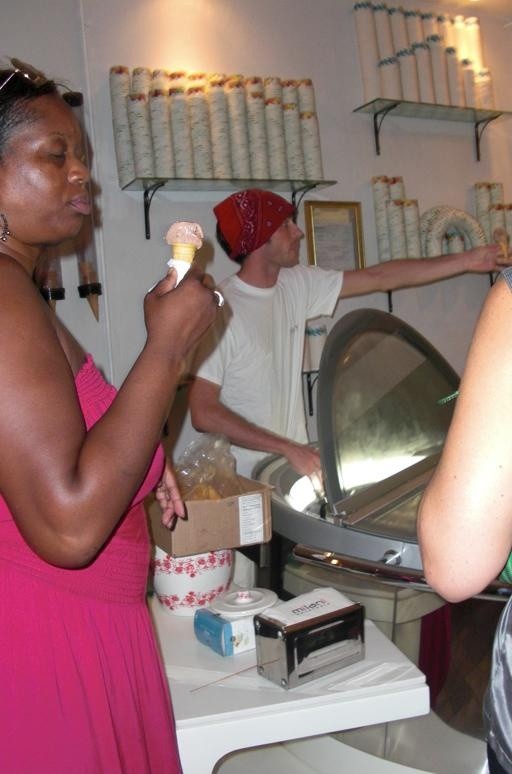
[1,55,225,774]
[413,262,512,773]
[187,185,512,592]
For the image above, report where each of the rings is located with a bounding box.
[213,289,224,307]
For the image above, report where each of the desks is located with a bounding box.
[142,594,438,773]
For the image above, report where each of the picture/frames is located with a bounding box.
[305,197,365,276]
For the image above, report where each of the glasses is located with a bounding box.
[2,57,46,92]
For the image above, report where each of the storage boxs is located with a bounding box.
[145,471,276,562]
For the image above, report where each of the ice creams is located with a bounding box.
[493,227,509,259]
[164,218,206,288]
